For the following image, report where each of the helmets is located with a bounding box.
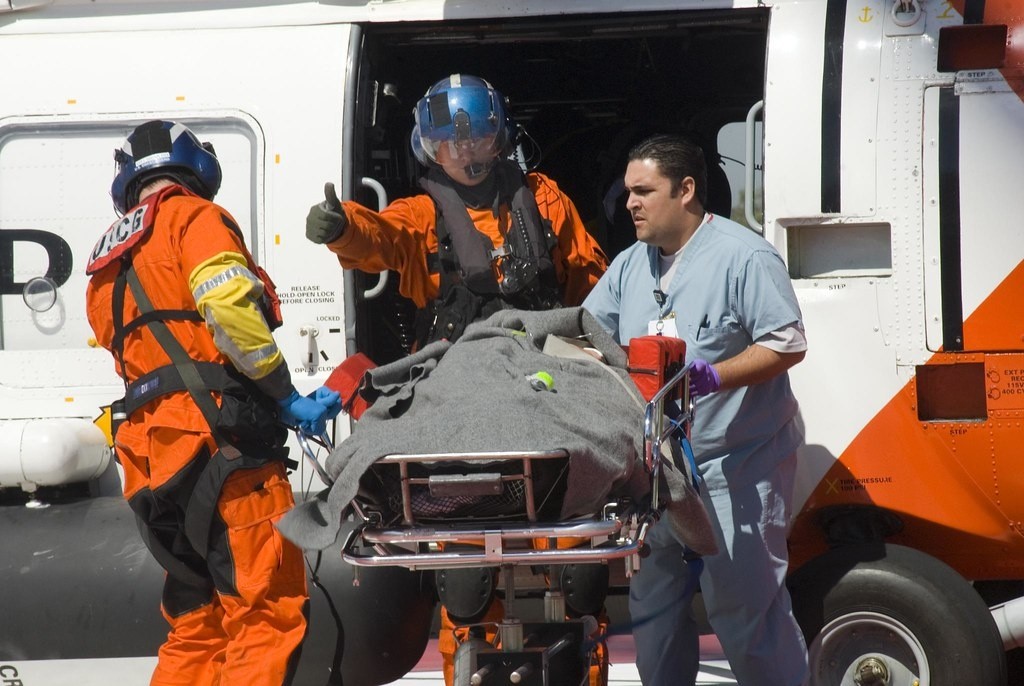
[415,74,498,141]
[110,119,222,214]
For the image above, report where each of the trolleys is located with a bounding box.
[295,332,696,686]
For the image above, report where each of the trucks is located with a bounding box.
[0,0,1024,685]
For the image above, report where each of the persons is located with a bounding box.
[305,74,613,686]
[580,134,812,686]
[86,119,343,686]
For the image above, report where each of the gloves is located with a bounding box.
[687,359,720,399]
[306,386,341,419]
[305,182,349,244]
[278,390,327,436]
